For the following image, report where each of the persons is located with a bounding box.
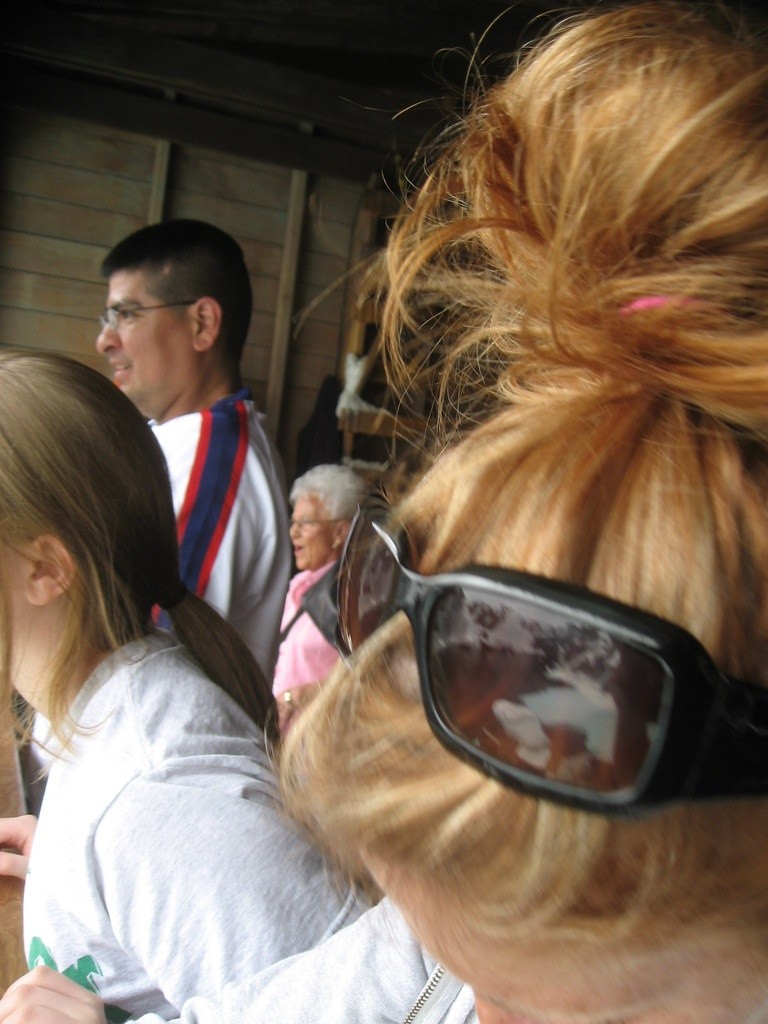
[0,0,768,1024]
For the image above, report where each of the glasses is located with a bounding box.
[288,517,337,531]
[98,302,190,324]
[333,497,766,812]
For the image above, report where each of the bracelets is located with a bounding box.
[283,687,301,718]
